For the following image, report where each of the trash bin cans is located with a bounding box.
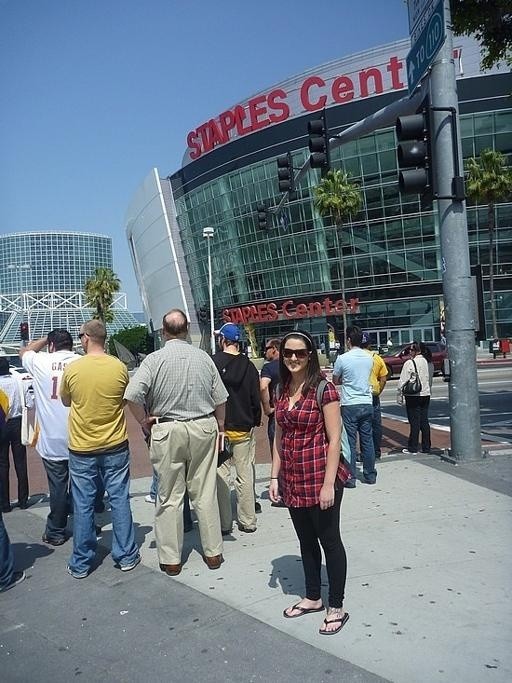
[489,339,510,359]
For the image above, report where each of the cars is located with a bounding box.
[9,366,36,399]
[377,341,448,380]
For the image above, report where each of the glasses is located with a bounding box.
[262,346,274,353]
[282,348,312,358]
[76,333,89,340]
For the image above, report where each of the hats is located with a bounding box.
[213,322,240,342]
[361,332,370,343]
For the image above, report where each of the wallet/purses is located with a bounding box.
[217,436,231,468]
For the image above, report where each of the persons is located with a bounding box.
[259,326,432,507]
[1,307,261,591]
[267,331,350,635]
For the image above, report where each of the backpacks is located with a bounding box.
[275,379,352,466]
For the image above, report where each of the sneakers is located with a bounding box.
[184,498,292,537]
[121,552,141,571]
[67,563,89,579]
[0,571,26,591]
[144,495,156,503]
[96,524,101,536]
[42,533,65,545]
[402,449,417,456]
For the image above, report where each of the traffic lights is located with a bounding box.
[257,200,269,233]
[21,322,29,340]
[277,149,294,195]
[307,106,331,177]
[395,105,435,197]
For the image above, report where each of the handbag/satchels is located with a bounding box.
[21,406,34,446]
[402,359,422,394]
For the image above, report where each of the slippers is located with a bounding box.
[284,602,325,618]
[319,612,349,635]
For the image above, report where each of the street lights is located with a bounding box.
[202,227,215,356]
[7,264,31,348]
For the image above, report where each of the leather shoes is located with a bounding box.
[159,562,182,576]
[203,552,223,570]
